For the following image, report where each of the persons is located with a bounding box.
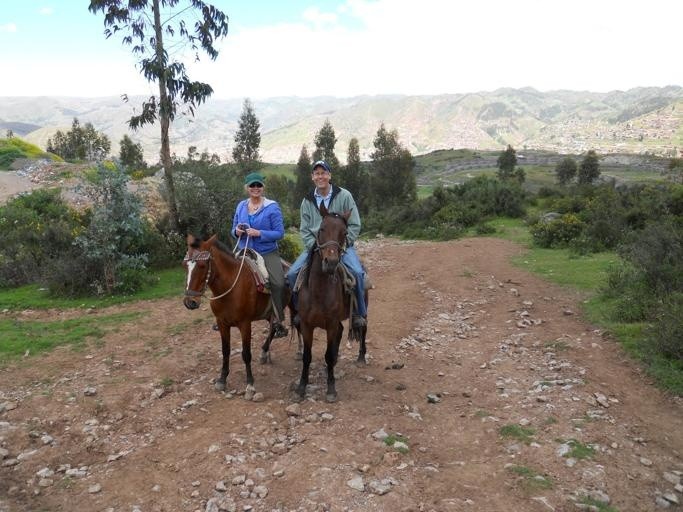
[280,159,370,332]
[208,172,290,339]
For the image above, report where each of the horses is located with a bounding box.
[291,199,369,403]
[182,230,305,401]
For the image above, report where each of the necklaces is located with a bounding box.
[252,206,258,210]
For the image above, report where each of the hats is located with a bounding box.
[245,173,265,188]
[312,160,331,172]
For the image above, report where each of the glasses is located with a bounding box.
[247,183,263,188]
